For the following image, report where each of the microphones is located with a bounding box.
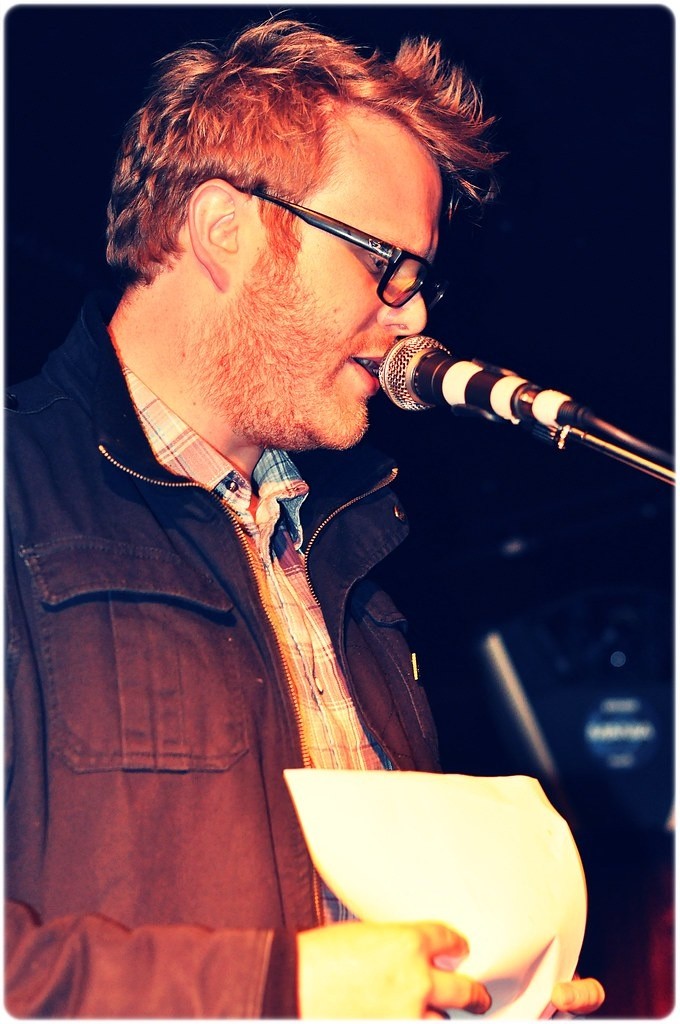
[379,335,591,427]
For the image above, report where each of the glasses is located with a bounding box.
[234,185,449,311]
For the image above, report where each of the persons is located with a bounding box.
[5,22,606,1020]
